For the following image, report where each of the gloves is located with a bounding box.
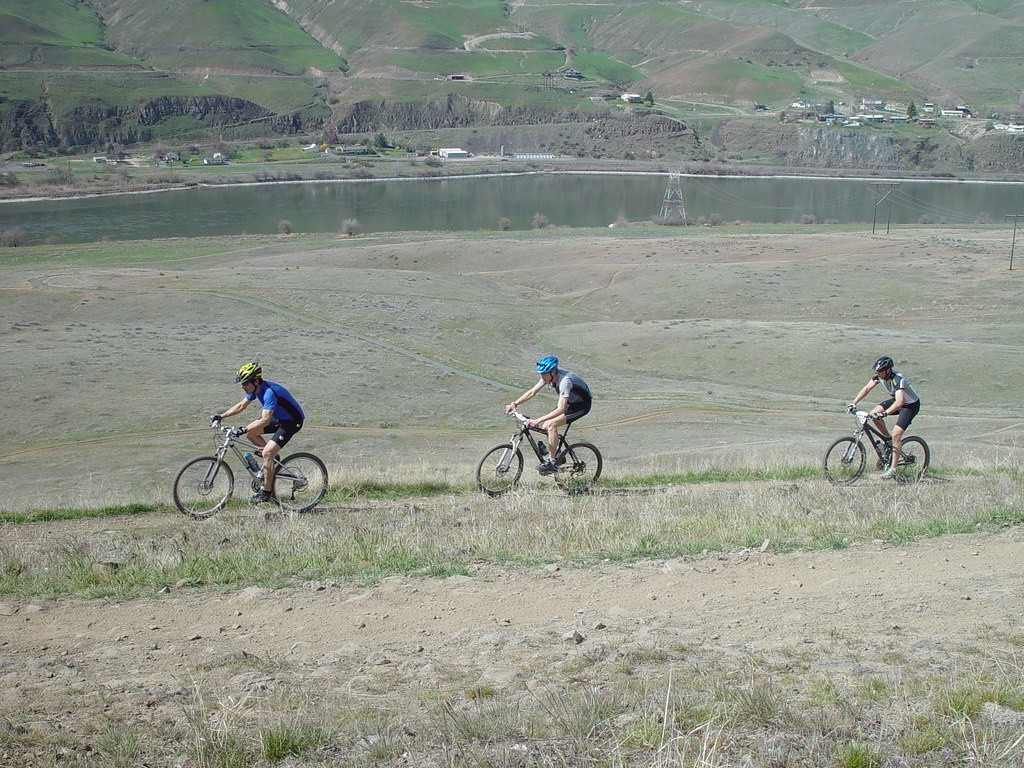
[211,416,222,423]
[236,427,247,438]
[846,402,856,413]
[874,411,887,419]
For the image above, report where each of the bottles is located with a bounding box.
[244,452,260,472]
[876,440,885,456]
[538,441,548,456]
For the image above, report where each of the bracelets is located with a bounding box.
[512,402,517,407]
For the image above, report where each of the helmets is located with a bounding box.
[536,356,558,373]
[872,356,894,371]
[235,362,261,383]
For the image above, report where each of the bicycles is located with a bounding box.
[475,409,603,495]
[172,421,328,514]
[821,402,931,486]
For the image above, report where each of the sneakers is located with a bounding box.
[535,459,558,471]
[273,454,280,463]
[548,455,566,464]
[880,469,896,479]
[884,440,892,447]
[253,490,271,502]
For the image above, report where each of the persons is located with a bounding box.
[506,356,592,471]
[847,355,920,479]
[211,362,305,504]
